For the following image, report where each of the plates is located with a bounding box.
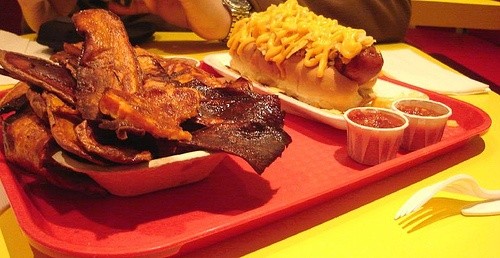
[204,52,429,131]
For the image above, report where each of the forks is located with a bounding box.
[395,175,500,220]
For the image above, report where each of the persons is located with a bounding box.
[17,0,412,45]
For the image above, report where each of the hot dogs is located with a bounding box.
[227,0,384,111]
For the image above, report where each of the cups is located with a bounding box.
[392,98,452,151]
[344,107,408,166]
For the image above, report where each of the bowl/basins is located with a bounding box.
[50,145,226,198]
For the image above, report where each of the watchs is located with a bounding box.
[222,0,256,42]
